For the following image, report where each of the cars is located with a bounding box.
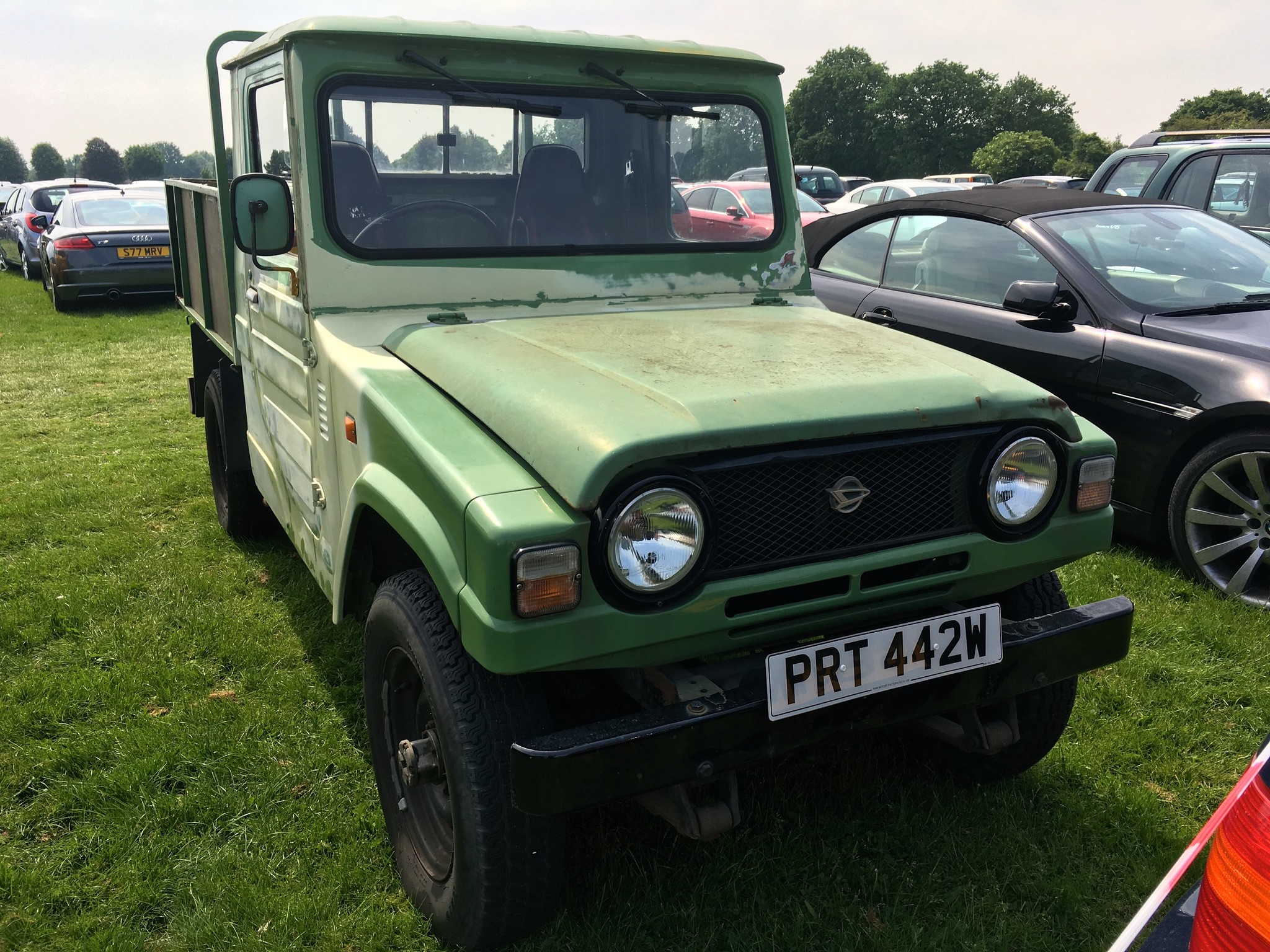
[30,188,175,314]
[669,164,1256,240]
[0,176,171,281]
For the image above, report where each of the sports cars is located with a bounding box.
[800,184,1270,613]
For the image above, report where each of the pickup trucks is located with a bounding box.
[162,14,1139,951]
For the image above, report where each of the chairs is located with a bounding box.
[330,139,395,248]
[506,144,603,246]
[915,228,1002,304]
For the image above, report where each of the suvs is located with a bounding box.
[1082,129,1270,245]
